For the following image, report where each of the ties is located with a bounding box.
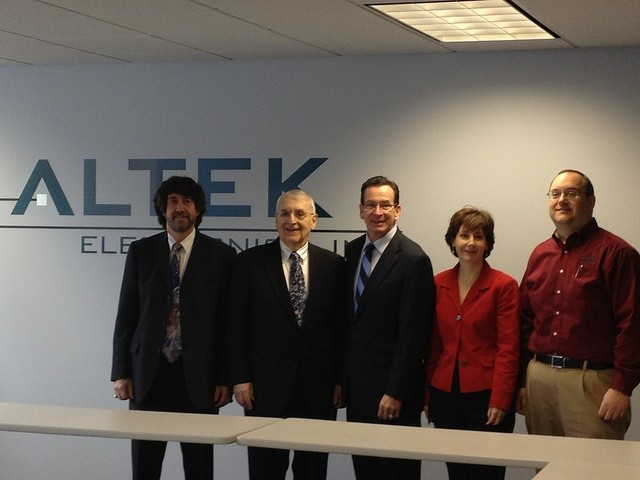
[288,250,308,326]
[162,243,184,366]
[354,243,374,316]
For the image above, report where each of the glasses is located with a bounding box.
[546,189,587,200]
[275,211,318,220]
[361,204,397,211]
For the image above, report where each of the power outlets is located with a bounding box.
[36,193,47,207]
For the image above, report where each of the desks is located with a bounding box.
[0,401,284,445]
[236,417,640,480]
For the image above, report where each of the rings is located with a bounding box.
[388,415,395,420]
[114,394,117,398]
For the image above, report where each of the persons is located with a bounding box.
[230,187,347,480]
[516,168,639,474]
[347,174,433,480]
[423,204,527,479]
[111,175,239,480]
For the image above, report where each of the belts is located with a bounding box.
[533,352,614,370]
